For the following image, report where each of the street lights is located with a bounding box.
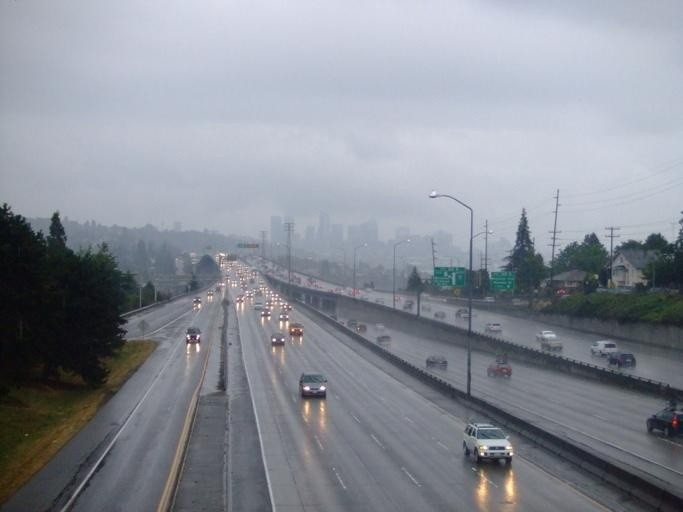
[351,243,368,297]
[139,265,155,308]
[427,191,493,395]
[392,240,410,310]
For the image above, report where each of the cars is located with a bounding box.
[535,330,556,340]
[425,354,447,369]
[309,321,394,346]
[591,341,617,355]
[540,337,563,352]
[396,296,505,335]
[191,253,385,320]
[270,332,285,346]
[487,360,512,377]
[298,369,327,398]
[289,322,303,336]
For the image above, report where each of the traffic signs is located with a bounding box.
[432,266,466,288]
[489,271,515,291]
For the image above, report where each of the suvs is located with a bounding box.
[645,406,682,437]
[462,421,513,464]
[185,327,201,343]
[606,350,635,369]
